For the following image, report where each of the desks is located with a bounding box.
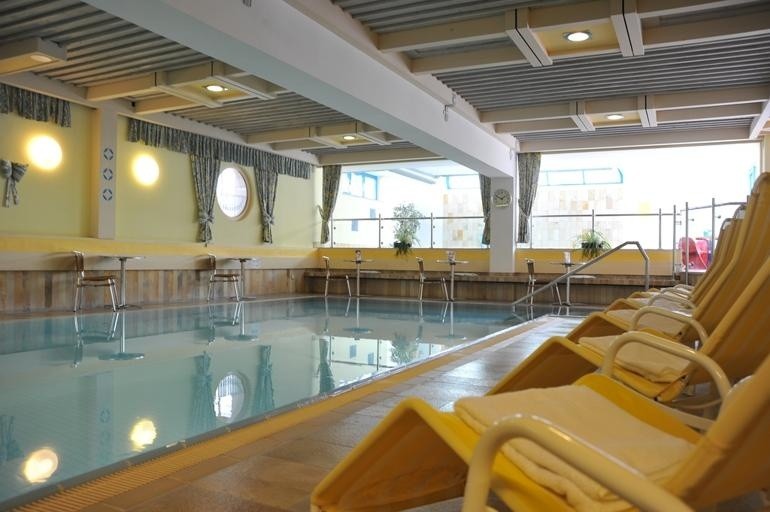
[99,253,143,311]
[226,256,258,300]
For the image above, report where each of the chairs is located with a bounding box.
[306,171,770,511]
[70,248,118,314]
[206,252,240,303]
